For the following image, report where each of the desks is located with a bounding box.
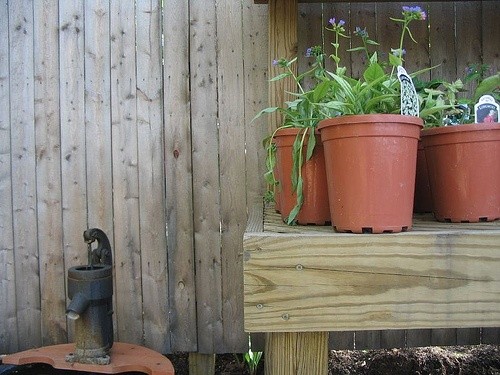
[242,204,500,375]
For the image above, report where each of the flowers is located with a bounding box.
[248,5,500,123]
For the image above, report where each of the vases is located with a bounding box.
[317,114,425,236]
[415,127,435,213]
[423,123,500,224]
[273,160,281,213]
[272,127,330,226]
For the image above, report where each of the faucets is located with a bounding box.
[61,225,114,366]
